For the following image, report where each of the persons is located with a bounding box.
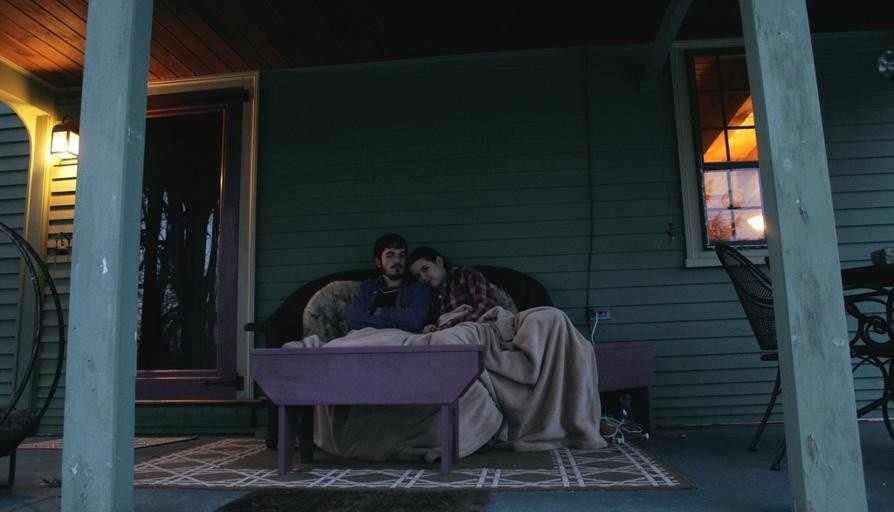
[346,234,431,333]
[409,246,497,333]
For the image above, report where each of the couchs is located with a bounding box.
[242,263,565,465]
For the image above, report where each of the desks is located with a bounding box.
[839,263,891,441]
[591,340,653,431]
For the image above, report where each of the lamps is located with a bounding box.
[50,114,79,160]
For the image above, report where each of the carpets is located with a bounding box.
[218,485,490,511]
[19,425,197,450]
[133,434,696,489]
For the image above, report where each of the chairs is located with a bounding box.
[714,241,893,475]
